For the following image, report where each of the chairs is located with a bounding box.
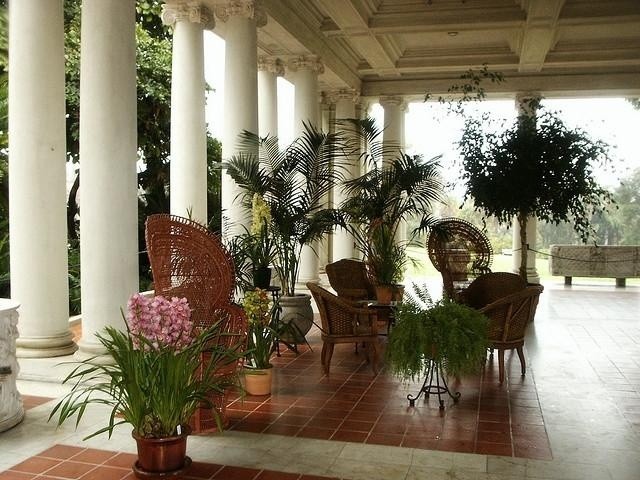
[464,271,543,385]
[428,216,494,300]
[138,211,252,434]
[325,257,385,352]
[299,281,378,376]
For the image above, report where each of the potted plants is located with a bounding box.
[211,127,349,340]
[338,115,448,304]
[430,68,630,325]
[383,285,492,379]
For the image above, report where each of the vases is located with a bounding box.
[252,267,273,289]
[130,423,192,480]
[243,362,275,396]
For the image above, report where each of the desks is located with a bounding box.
[350,298,406,363]
[548,244,640,288]
[244,285,282,357]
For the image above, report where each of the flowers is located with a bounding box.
[246,194,277,269]
[234,285,275,369]
[46,288,250,436]
